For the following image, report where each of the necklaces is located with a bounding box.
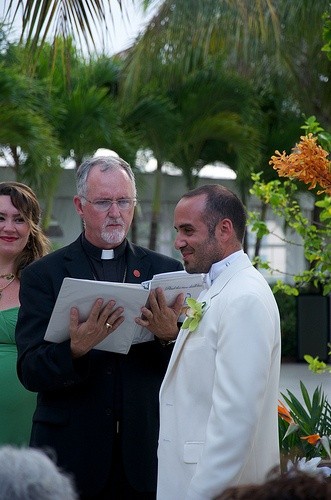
[82,238,127,283]
[0,272,16,299]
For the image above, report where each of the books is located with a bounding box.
[44,271,212,354]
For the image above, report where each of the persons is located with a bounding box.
[0,181,56,449]
[0,441,79,500]
[156,184,281,500]
[212,468,331,500]
[15,156,184,500]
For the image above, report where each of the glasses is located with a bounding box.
[82,195,137,212]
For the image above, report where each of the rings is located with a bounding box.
[105,322,113,330]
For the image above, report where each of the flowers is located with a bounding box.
[182,297,206,332]
[277,380,331,480]
